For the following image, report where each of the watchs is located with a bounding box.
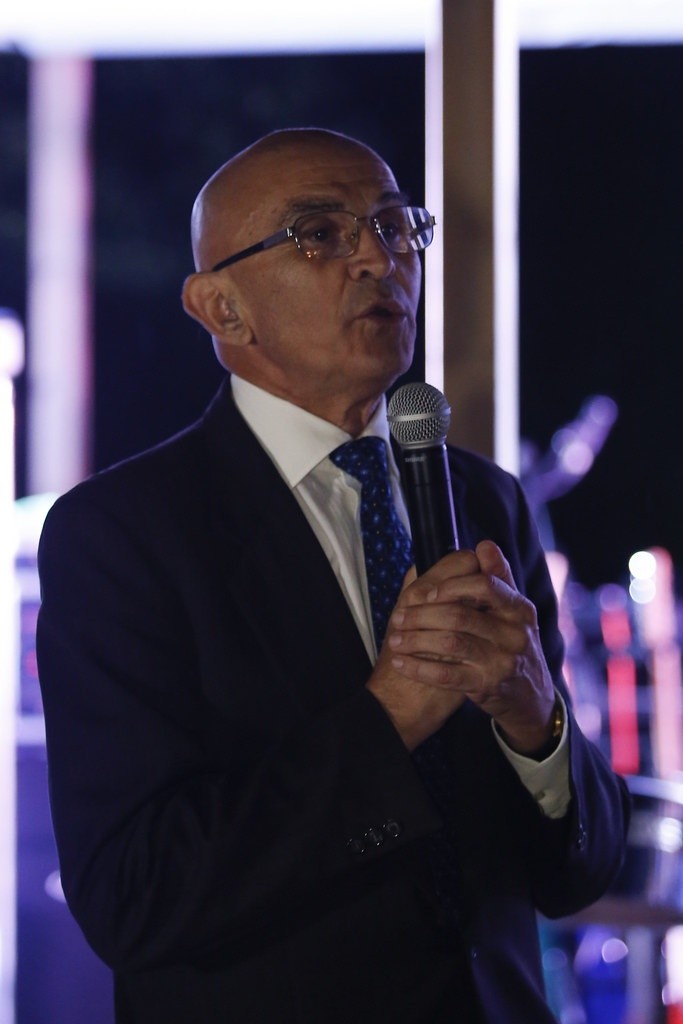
[527,703,562,761]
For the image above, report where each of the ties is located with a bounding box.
[329,435,416,662]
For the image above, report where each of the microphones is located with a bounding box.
[384,380,461,576]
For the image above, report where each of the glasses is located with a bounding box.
[210,206,437,271]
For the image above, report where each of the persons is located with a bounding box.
[35,127,629,1024]
